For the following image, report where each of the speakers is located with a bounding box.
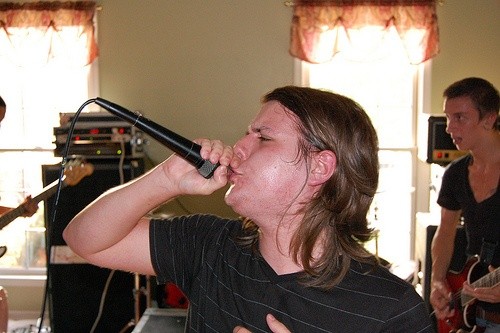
[41,161,146,333]
[424,225,467,303]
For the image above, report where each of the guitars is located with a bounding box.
[429,254,500,333]
[0,159,96,333]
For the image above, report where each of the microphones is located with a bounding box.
[95,97,233,180]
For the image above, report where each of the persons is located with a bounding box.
[0,97,39,333]
[429,77,500,333]
[63,86,434,333]
